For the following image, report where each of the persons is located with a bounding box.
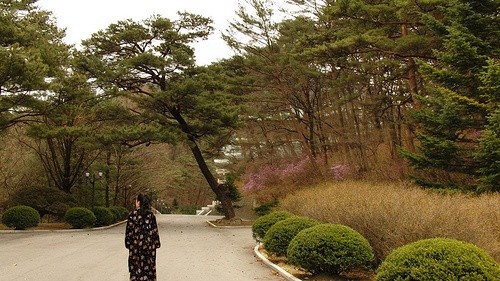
[124,193,162,281]
